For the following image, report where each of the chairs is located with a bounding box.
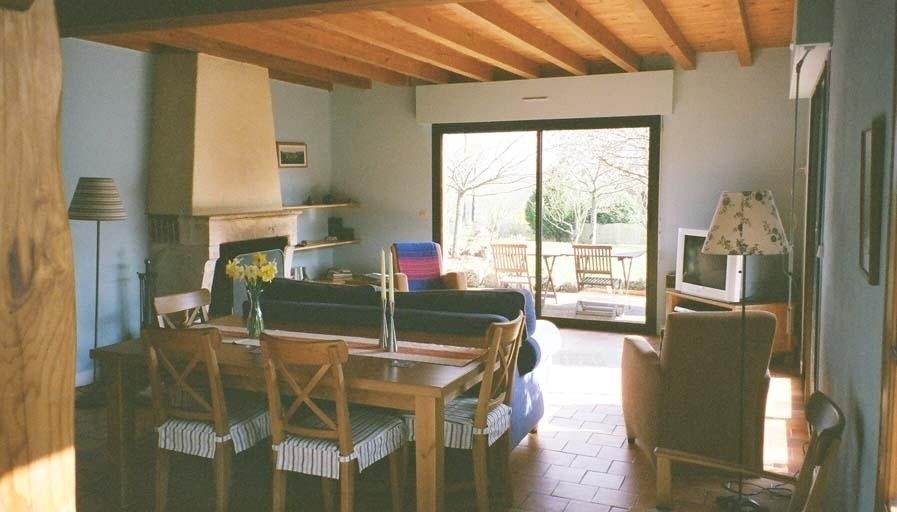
[621,311,778,469]
[391,242,467,292]
[572,245,623,319]
[490,244,535,297]
[653,391,845,512]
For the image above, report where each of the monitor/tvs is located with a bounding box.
[675,227,776,304]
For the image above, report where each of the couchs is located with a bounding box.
[242,279,561,452]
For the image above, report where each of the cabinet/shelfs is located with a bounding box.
[283,203,359,252]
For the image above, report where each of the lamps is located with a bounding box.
[68,177,127,409]
[701,191,792,511]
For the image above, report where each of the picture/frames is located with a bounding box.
[277,142,308,168]
[859,123,884,285]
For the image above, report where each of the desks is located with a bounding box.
[90,315,500,512]
[665,288,789,356]
[513,242,646,309]
